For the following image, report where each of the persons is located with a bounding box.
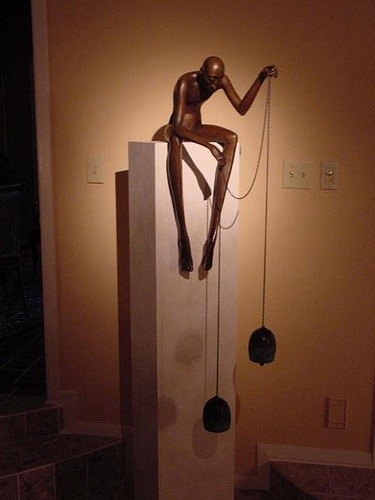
[164,55,279,270]
